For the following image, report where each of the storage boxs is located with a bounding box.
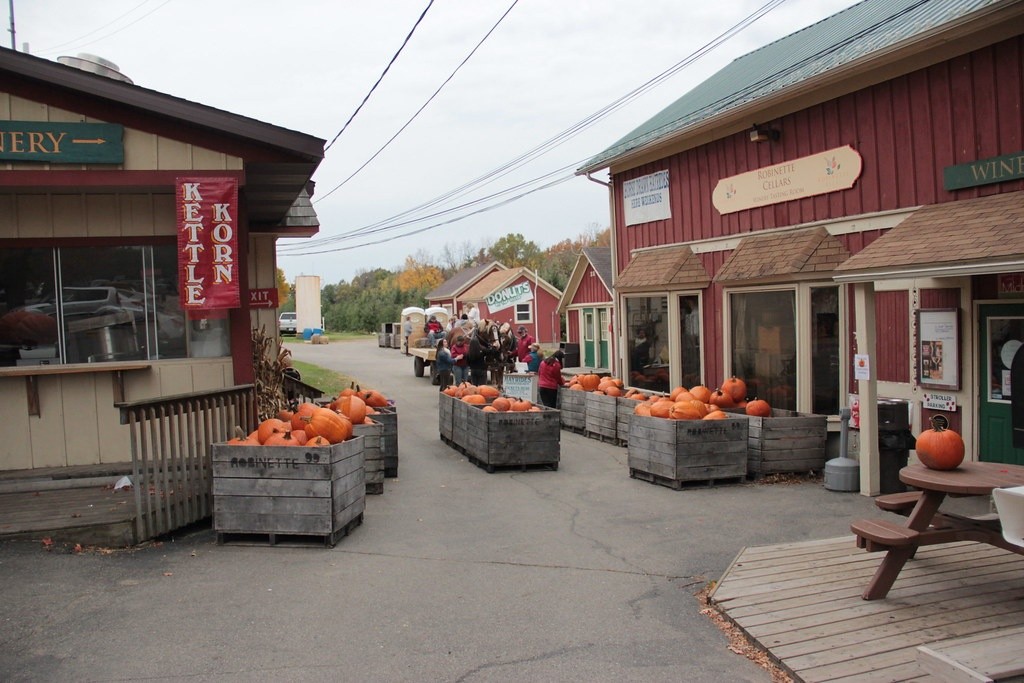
[206,365,831,551]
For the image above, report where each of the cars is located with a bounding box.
[279,312,298,333]
[13,278,185,346]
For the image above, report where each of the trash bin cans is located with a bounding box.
[877,401,916,493]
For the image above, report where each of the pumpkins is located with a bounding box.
[443,380,543,413]
[226,381,386,446]
[916,416,966,470]
[564,370,771,420]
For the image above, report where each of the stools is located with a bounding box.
[875,491,934,515]
[849,516,919,552]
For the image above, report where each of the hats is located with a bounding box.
[430,316,436,320]
[516,326,528,333]
[455,334,464,342]
[528,343,541,350]
[460,314,468,319]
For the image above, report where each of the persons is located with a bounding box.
[450,336,470,388]
[404,301,481,357]
[435,339,464,393]
[508,325,536,363]
[631,329,651,369]
[537,350,566,409]
[468,338,489,386]
[521,342,545,373]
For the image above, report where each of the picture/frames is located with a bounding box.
[914,307,962,391]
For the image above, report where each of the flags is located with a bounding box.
[174,176,243,310]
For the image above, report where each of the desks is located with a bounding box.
[862,457,1024,600]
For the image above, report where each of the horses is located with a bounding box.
[486,319,517,386]
[446,320,500,386]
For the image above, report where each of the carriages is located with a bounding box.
[399,309,515,391]
